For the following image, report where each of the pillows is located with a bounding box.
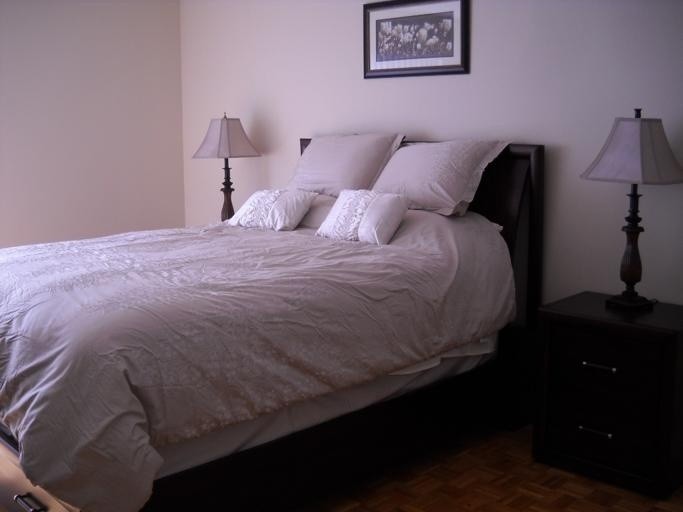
[228,130,511,246]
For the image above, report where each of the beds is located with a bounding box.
[0,138,545,511]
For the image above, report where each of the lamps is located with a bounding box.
[579,107,682,319]
[191,112,262,222]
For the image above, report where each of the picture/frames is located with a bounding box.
[364,0,470,79]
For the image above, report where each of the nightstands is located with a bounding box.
[536,291,683,499]
